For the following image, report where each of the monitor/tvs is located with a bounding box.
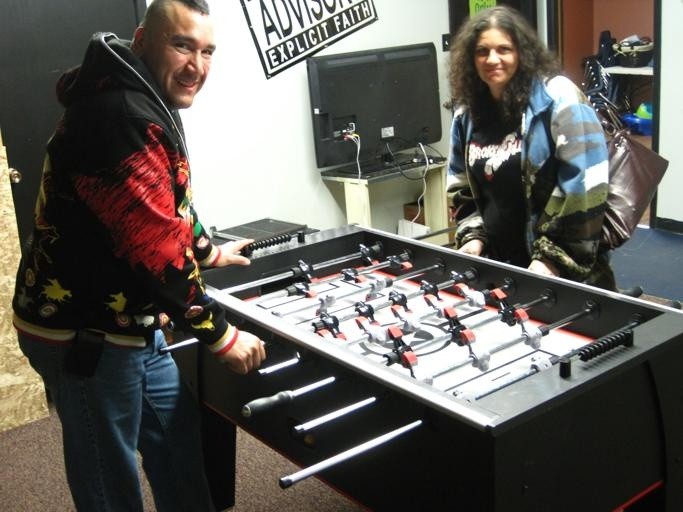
[306,42,447,175]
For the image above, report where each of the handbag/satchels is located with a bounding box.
[599,129,669,248]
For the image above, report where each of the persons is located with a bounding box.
[444,5,619,295]
[11,0,267,512]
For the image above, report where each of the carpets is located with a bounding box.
[0,412,365,512]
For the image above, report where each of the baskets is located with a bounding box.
[612,37,654,67]
